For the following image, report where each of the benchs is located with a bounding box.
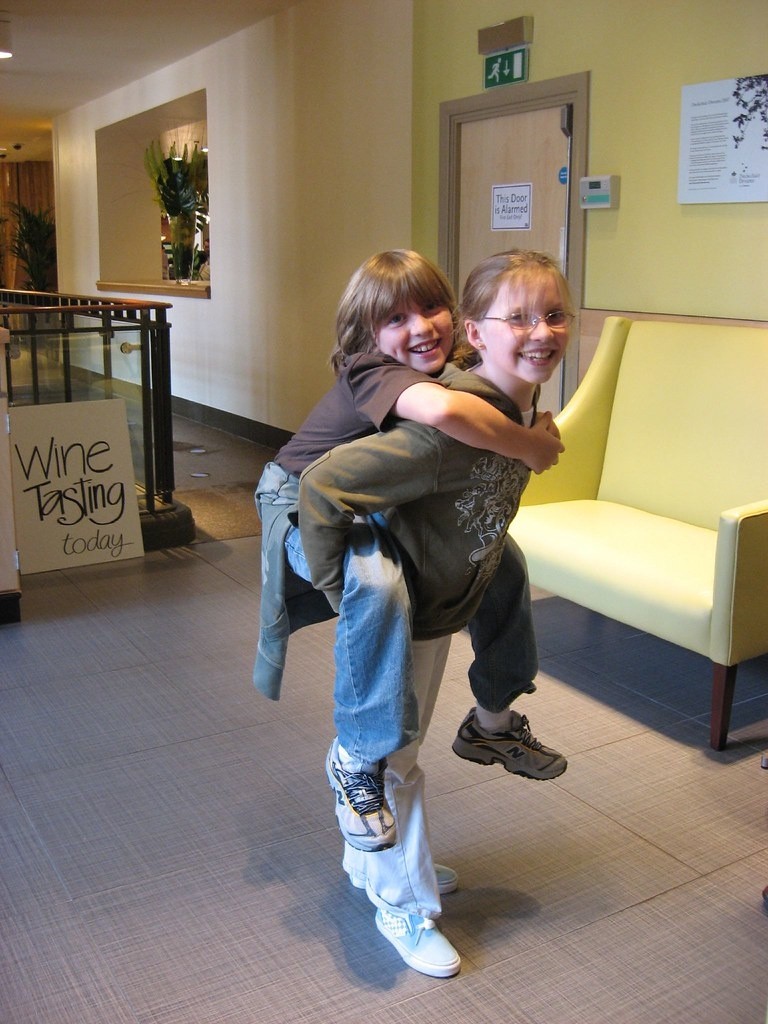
[509,315,767,750]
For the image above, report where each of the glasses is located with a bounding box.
[484,309,576,330]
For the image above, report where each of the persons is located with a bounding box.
[297,249,578,977]
[262,250,569,853]
[197,239,210,281]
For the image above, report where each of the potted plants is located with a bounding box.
[144,126,211,285]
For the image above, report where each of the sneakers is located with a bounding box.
[374,907,461,978]
[452,707,567,781]
[351,863,459,895]
[325,735,397,852]
